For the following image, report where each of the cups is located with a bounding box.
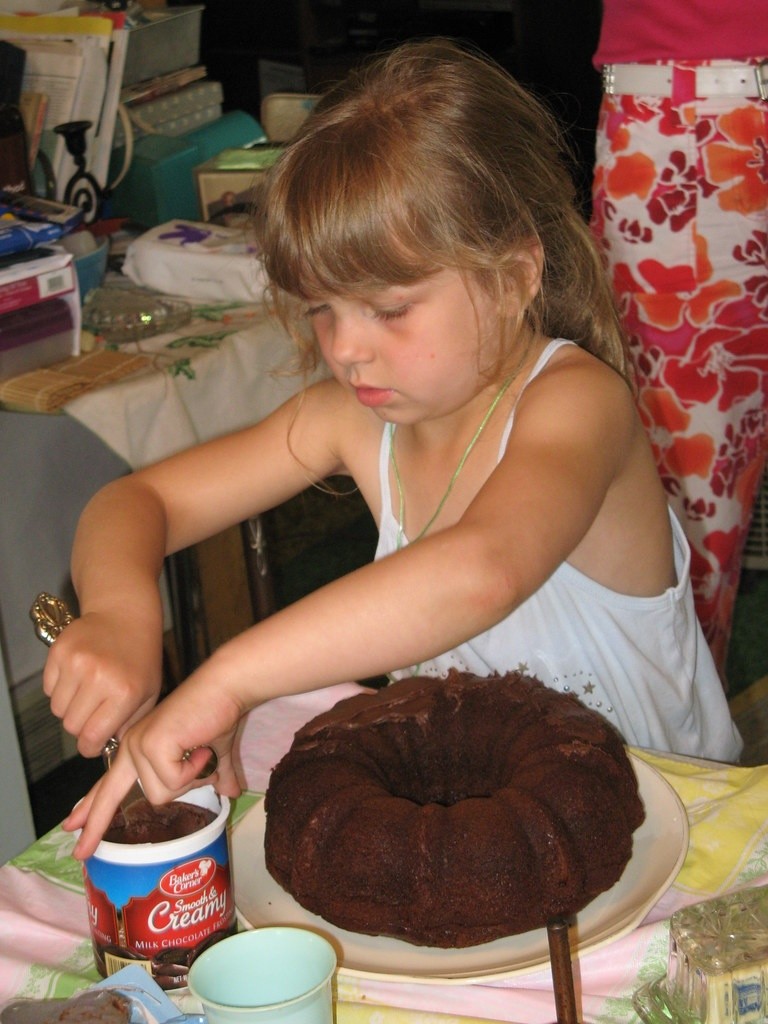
[186,929,337,1024]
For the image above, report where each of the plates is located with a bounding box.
[233,747,690,981]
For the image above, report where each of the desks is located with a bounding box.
[0,290,338,871]
[1,675,768,1024]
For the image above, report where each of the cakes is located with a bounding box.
[261,668,645,949]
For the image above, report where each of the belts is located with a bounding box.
[602,63,768,100]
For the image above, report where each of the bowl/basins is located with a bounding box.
[73,234,109,302]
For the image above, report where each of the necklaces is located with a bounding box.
[389,346,526,550]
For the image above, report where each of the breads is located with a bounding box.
[57,990,133,1024]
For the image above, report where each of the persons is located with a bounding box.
[585,2,768,697]
[42,37,748,860]
